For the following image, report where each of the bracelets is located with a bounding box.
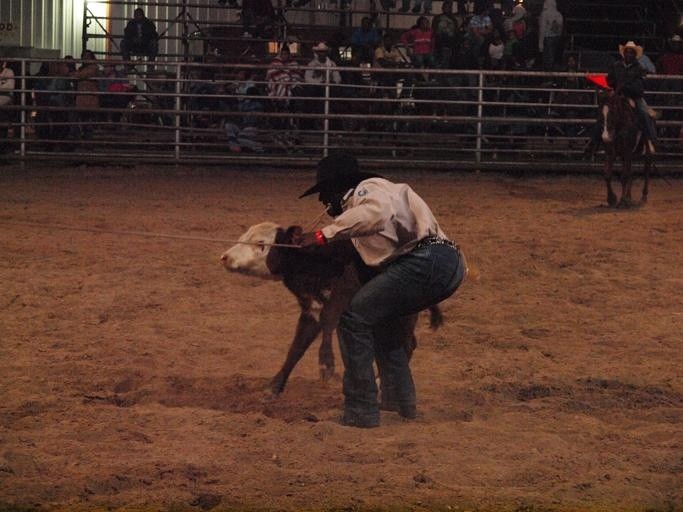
[315,230,326,244]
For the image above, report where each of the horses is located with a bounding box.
[596,85,658,210]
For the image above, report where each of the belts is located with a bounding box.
[416,236,458,250]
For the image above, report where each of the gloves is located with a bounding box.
[297,230,328,253]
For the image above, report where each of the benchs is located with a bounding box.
[81,0,683,109]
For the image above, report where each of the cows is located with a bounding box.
[221,221,444,401]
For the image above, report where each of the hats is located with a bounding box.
[618,40,643,60]
[312,41,332,52]
[297,152,387,199]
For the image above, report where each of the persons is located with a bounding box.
[639,34,682,161]
[296,148,470,432]
[1,51,133,153]
[601,41,663,161]
[121,1,586,161]
